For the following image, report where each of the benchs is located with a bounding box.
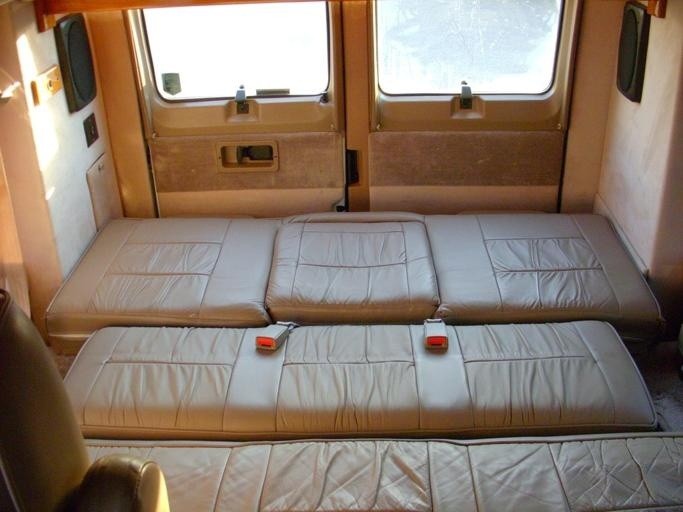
[41,210,666,347]
[54,319,663,441]
[78,425,682,512]
